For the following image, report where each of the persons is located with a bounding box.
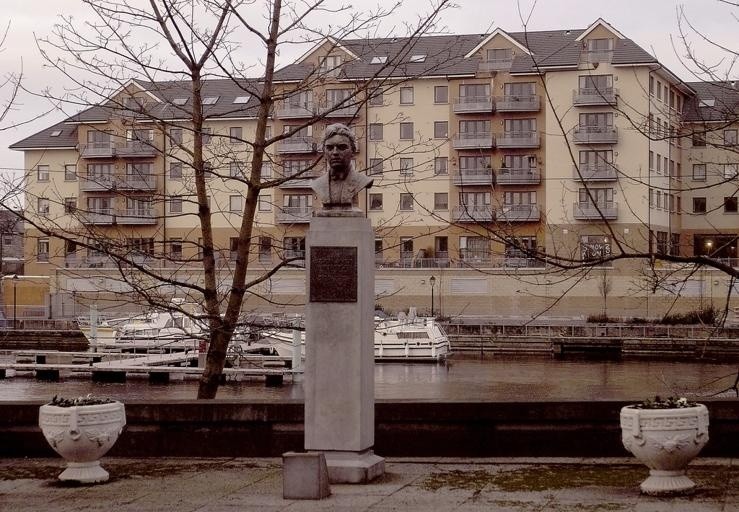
[308,125,375,215]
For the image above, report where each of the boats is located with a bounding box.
[0,311,455,365]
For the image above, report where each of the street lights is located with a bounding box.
[11,273,20,329]
[428,274,437,315]
